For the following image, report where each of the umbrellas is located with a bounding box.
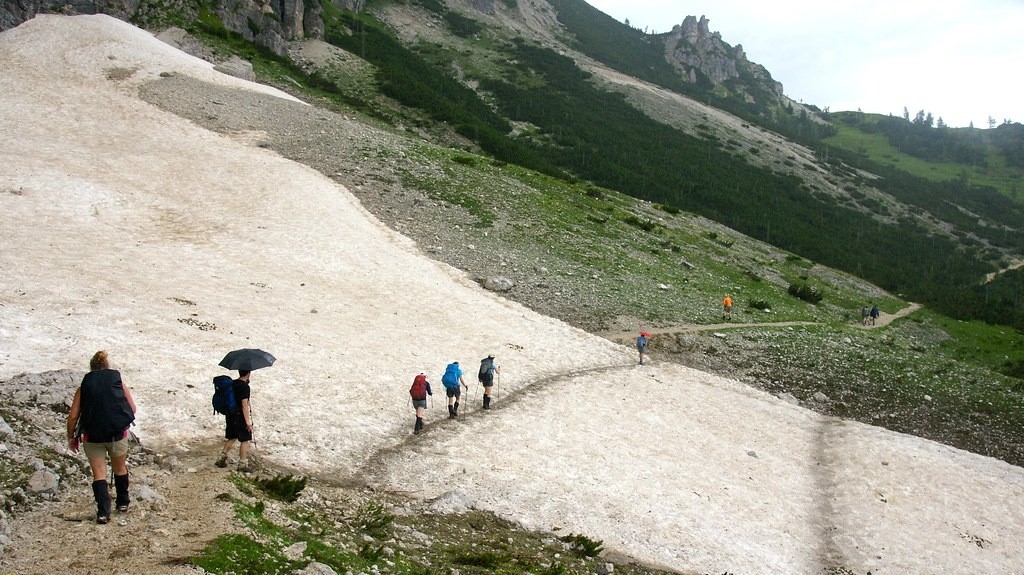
[217,348,277,379]
[639,331,653,338]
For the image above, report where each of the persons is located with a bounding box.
[637,334,648,364]
[67,349,136,523]
[862,306,871,326]
[870,306,880,325]
[722,293,733,321]
[442,361,468,418]
[478,354,501,410]
[212,371,257,473]
[408,371,433,434]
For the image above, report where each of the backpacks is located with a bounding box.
[637,337,645,349]
[212,375,247,417]
[79,369,134,440]
[478,358,493,383]
[409,375,427,400]
[442,364,459,389]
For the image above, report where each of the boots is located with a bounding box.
[449,405,455,419]
[454,402,459,416]
[414,415,423,434]
[114,472,130,512]
[483,394,490,410]
[92,479,111,523]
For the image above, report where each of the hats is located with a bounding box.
[488,354,495,358]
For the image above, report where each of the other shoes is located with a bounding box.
[218,453,228,467]
[239,459,254,472]
